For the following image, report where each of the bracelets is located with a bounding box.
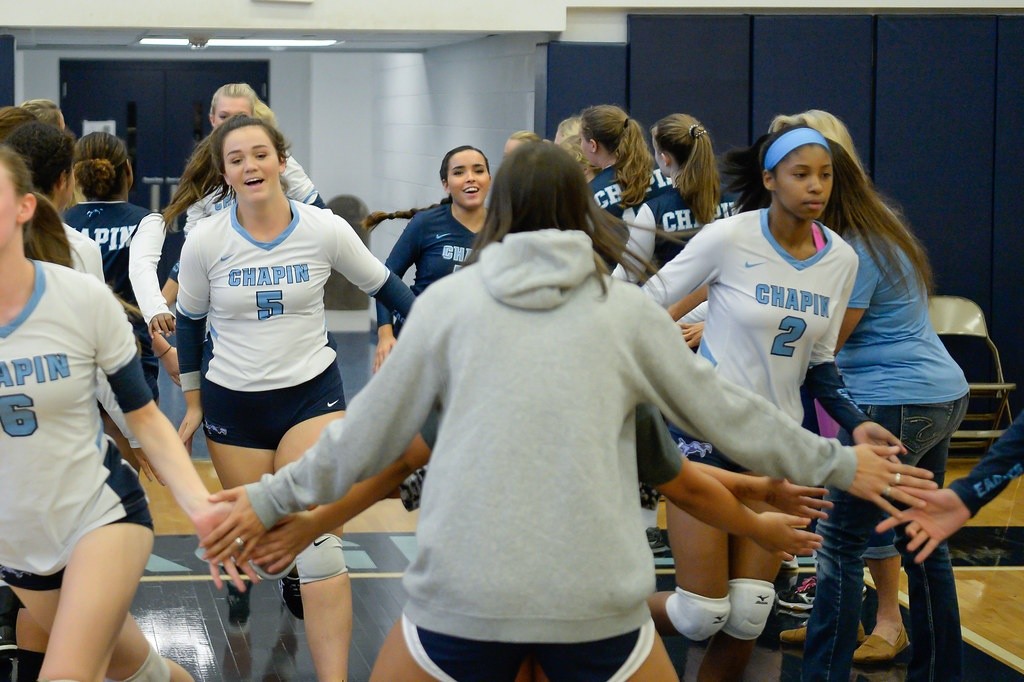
[157,345,173,359]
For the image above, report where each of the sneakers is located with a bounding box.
[776,576,818,610]
[280,575,304,621]
[225,580,253,626]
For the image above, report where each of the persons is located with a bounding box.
[176,113,418,681]
[0,144,269,682]
[768,109,965,682]
[612,113,743,285]
[162,84,325,305]
[362,145,491,365]
[199,139,938,681]
[875,409,1024,562]
[503,131,539,160]
[61,132,176,402]
[0,95,180,491]
[554,104,672,288]
[642,125,908,682]
[776,515,867,610]
[782,511,910,662]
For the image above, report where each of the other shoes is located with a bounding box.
[780,620,865,645]
[853,622,910,664]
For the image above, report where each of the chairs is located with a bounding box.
[927,295,1017,455]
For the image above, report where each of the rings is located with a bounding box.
[895,472,901,484]
[886,486,892,496]
[230,555,236,562]
[234,535,245,546]
